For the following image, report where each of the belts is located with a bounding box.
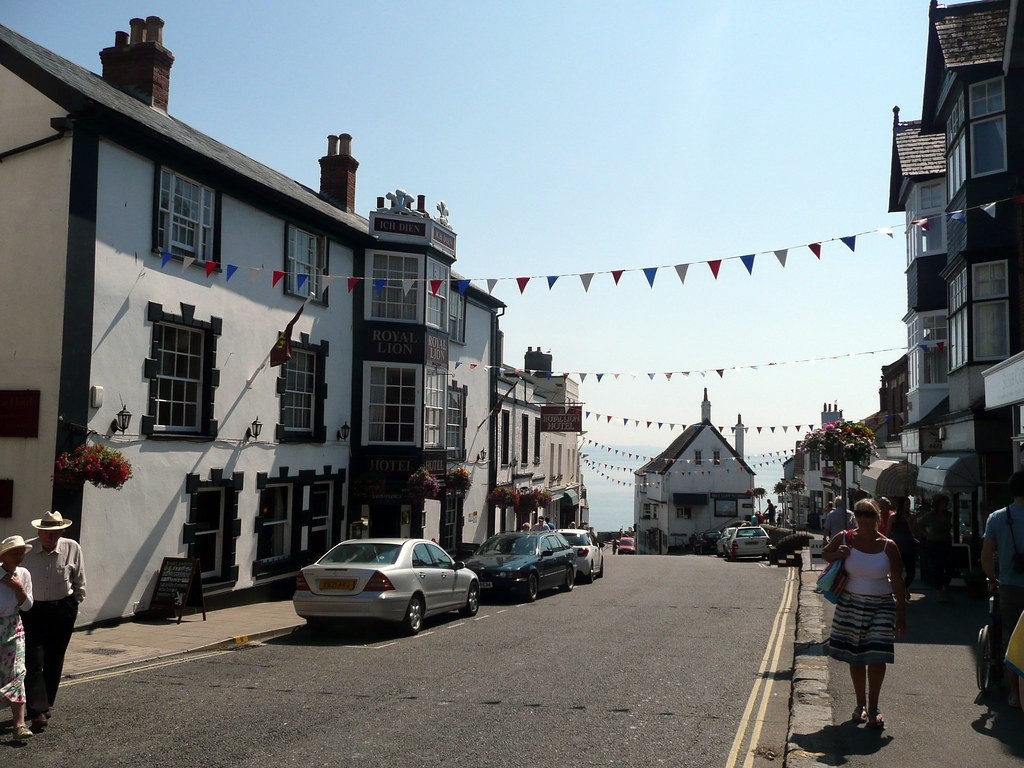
[34,600,63,607]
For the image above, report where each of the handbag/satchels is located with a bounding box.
[1011,553,1024,574]
[816,530,849,604]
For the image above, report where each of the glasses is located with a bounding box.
[854,510,876,518]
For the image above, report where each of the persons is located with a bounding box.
[20,511,87,728]
[822,498,906,729]
[569,521,595,538]
[982,472,1024,711]
[877,497,953,598]
[521,516,555,532]
[823,496,857,547]
[0,536,34,738]
[765,499,776,526]
[612,538,617,555]
[757,513,764,524]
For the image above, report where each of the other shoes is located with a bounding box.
[13,723,33,739]
[868,708,884,728]
[1008,694,1020,707]
[852,705,867,723]
[32,712,47,727]
[901,576,911,600]
[43,709,51,717]
[936,585,947,602]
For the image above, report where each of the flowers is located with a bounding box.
[409,466,441,504]
[350,470,388,499]
[443,467,472,498]
[752,487,768,500]
[520,493,540,511]
[50,441,134,491]
[485,485,521,509]
[785,477,808,495]
[538,489,554,509]
[800,420,880,482]
[773,482,788,494]
[744,489,755,499]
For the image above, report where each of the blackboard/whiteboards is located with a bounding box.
[150,558,206,610]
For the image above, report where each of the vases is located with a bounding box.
[353,495,377,505]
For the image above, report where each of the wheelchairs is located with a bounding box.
[976,578,1001,692]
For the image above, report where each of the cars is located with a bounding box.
[558,529,604,584]
[465,530,575,603]
[618,537,635,555]
[692,525,771,562]
[292,538,479,636]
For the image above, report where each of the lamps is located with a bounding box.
[110,403,133,435]
[246,416,263,440]
[477,448,487,461]
[336,420,351,442]
[532,456,540,465]
[509,456,518,468]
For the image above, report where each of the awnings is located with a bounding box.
[916,453,983,494]
[565,489,578,505]
[861,459,906,498]
[673,493,708,506]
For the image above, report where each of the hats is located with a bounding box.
[0,535,33,563]
[31,511,72,530]
